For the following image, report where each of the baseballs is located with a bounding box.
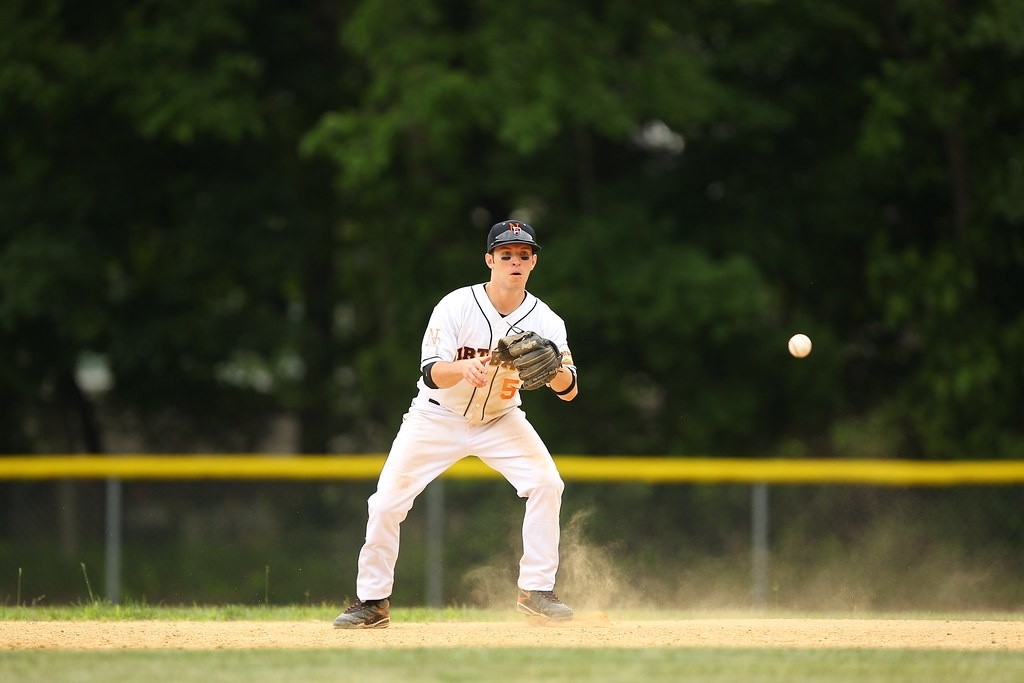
[788,333,813,359]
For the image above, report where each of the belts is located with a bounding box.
[429,399,440,406]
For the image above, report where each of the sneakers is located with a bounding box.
[516,587,573,621]
[334,596,390,629]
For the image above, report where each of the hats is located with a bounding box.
[487,219,541,254]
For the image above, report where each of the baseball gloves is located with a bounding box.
[497,330,563,391]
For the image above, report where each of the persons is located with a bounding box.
[335,221,578,627]
